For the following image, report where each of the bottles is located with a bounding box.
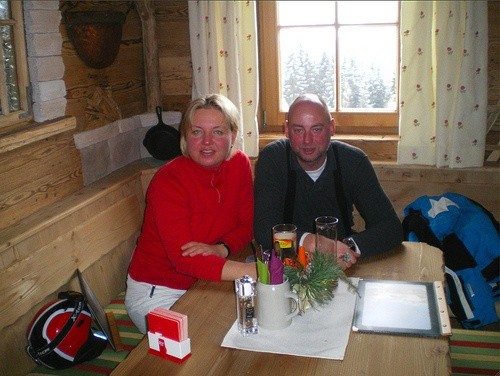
[235,275,257,335]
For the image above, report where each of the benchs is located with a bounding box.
[0,157,500,376]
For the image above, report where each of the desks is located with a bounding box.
[109,240,451,376]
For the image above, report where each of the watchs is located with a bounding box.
[343,238,356,252]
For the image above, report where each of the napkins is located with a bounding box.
[269,249,283,285]
[257,257,271,285]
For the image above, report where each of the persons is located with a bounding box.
[125,93,259,336]
[252,93,403,271]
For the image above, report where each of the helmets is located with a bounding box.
[25,292,109,370]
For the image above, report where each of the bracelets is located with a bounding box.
[216,241,230,259]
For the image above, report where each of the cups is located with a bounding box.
[314,216,338,267]
[273,224,298,264]
[255,274,300,330]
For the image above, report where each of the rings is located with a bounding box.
[343,253,352,262]
